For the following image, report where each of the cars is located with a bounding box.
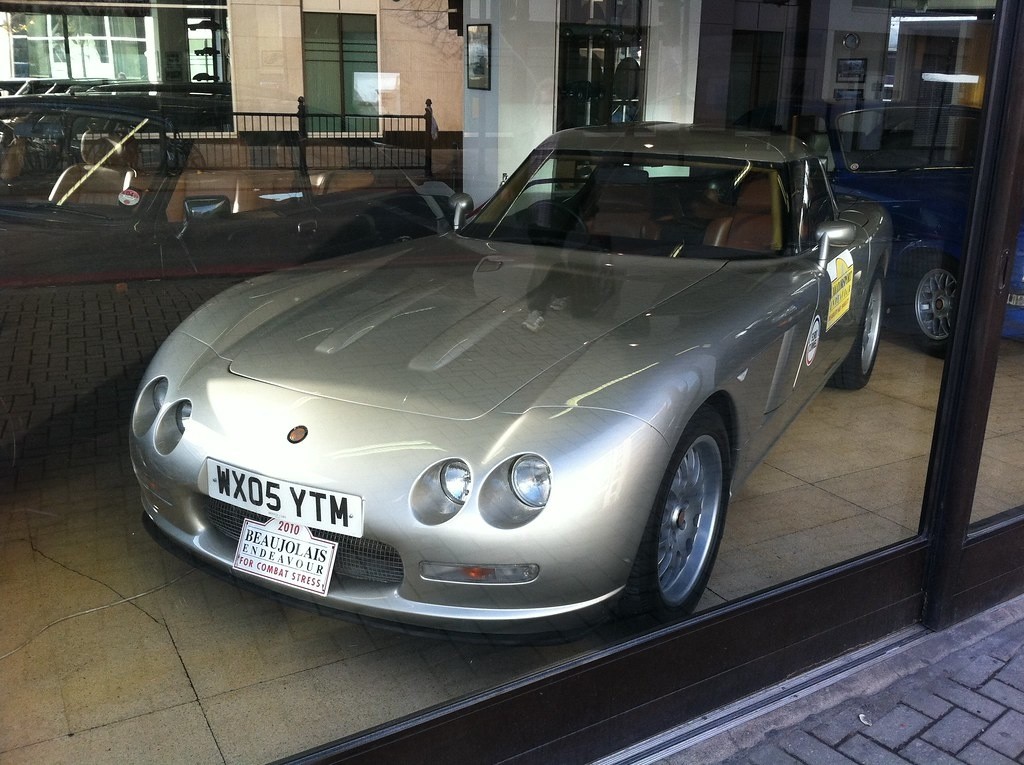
[1,79,465,291]
[716,96,1024,359]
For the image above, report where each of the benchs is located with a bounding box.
[143,132,376,207]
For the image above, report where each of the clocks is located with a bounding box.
[842,32,860,50]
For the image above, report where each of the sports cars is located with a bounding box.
[128,114,895,649]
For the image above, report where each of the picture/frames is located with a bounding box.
[466,24,492,92]
[833,88,864,100]
[872,83,882,92]
[835,58,868,83]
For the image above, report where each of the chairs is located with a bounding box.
[165,122,256,224]
[48,127,143,209]
[573,168,663,241]
[702,177,809,251]
[0,116,27,181]
